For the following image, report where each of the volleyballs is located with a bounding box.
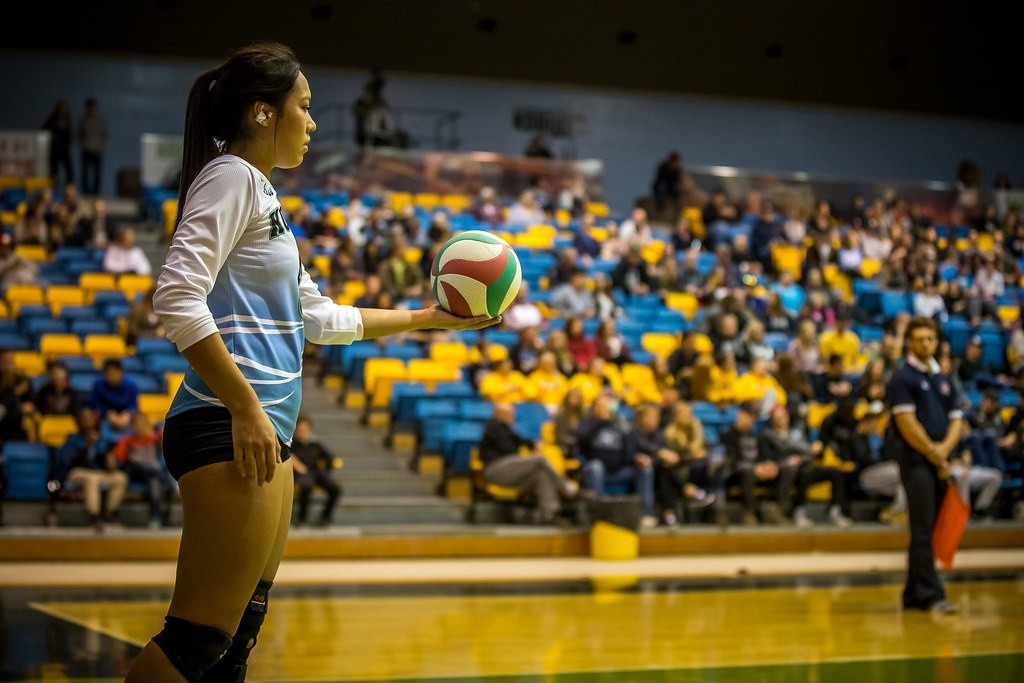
[430,229,523,319]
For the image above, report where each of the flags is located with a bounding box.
[930,483,970,568]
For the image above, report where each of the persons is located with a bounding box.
[0,186,186,526]
[886,318,966,616]
[43,99,75,186]
[77,96,106,196]
[288,417,342,525]
[525,134,553,186]
[280,162,1024,528]
[126,42,505,683]
[350,81,410,150]
[652,150,683,213]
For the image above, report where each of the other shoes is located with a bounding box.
[904,599,961,615]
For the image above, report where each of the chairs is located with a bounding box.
[0,176,1024,526]
